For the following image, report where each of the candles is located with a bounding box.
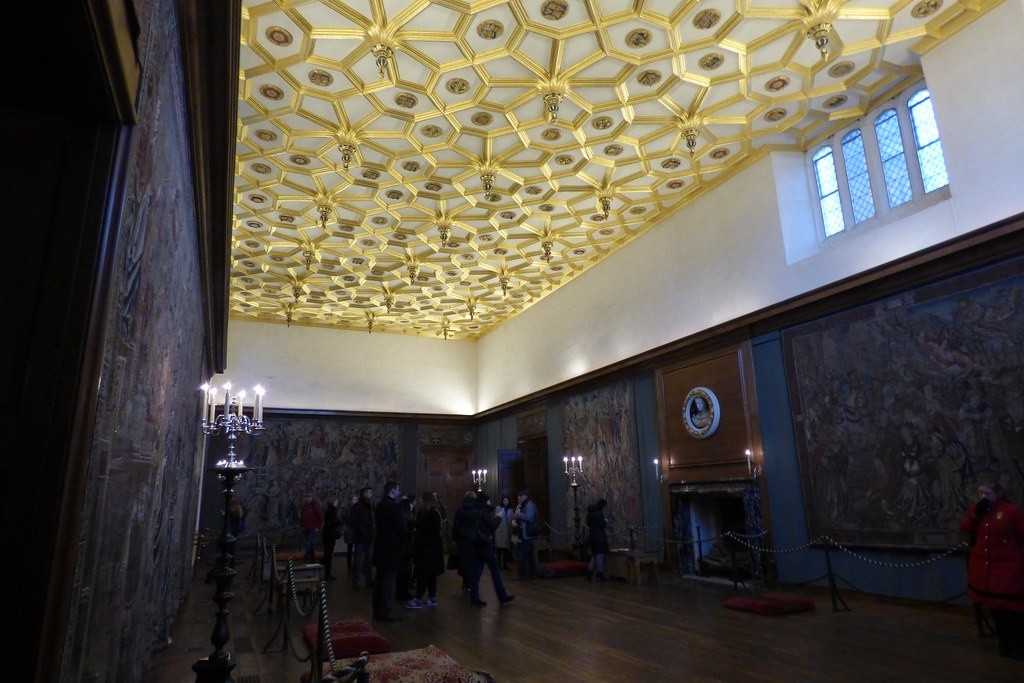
[564,456,584,475]
[654,458,658,479]
[746,450,751,474]
[199,380,265,423]
[472,469,487,483]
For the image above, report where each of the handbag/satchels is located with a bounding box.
[525,521,541,537]
[474,529,496,546]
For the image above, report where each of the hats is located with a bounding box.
[477,494,491,503]
[305,492,312,497]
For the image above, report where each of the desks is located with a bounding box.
[607,549,659,591]
[278,564,324,612]
[532,544,553,574]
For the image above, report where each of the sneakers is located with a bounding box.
[422,598,437,606]
[405,599,422,608]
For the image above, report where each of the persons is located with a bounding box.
[961,479,1024,661]
[350,487,374,590]
[342,495,359,577]
[494,495,513,570]
[300,493,320,565]
[510,488,537,581]
[471,495,514,605]
[452,490,477,590]
[585,499,610,583]
[690,397,710,431]
[320,497,341,581]
[371,481,443,621]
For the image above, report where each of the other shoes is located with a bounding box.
[587,572,593,580]
[503,563,511,571]
[499,596,514,605]
[512,576,526,581]
[528,573,536,579]
[373,608,402,621]
[325,576,336,581]
[471,599,487,607]
[596,574,609,581]
